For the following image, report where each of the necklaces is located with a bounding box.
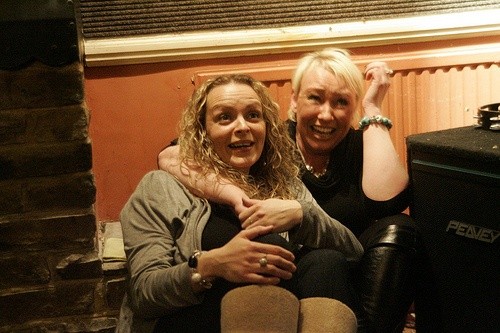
[297,143,329,177]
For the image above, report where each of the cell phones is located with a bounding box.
[55,253,83,273]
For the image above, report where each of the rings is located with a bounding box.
[248,217,253,224]
[384,67,392,75]
[260,252,267,267]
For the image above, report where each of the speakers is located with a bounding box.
[407,125,500,333]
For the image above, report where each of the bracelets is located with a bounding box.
[358,114,392,132]
[188,249,212,289]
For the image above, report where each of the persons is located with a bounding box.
[119,75,363,333]
[156,48,420,333]
